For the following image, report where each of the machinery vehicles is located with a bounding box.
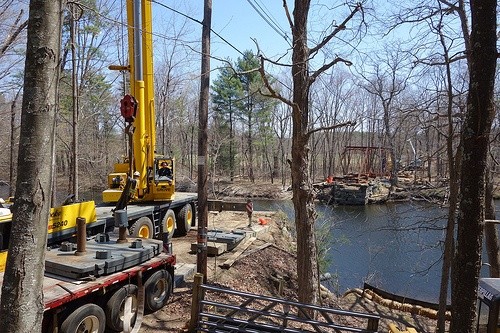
[0,210,177,333]
[0,0,199,275]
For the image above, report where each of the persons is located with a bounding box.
[118,171,141,203]
[246,200,254,226]
[158,163,172,178]
